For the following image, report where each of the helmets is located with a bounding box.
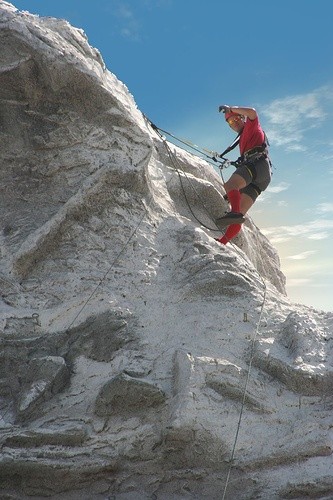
[225,106,240,120]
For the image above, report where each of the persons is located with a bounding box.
[214,105,272,246]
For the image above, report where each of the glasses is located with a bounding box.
[228,116,242,125]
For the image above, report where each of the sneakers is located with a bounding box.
[213,238,226,245]
[216,211,245,225]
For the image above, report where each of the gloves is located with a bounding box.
[219,105,230,113]
